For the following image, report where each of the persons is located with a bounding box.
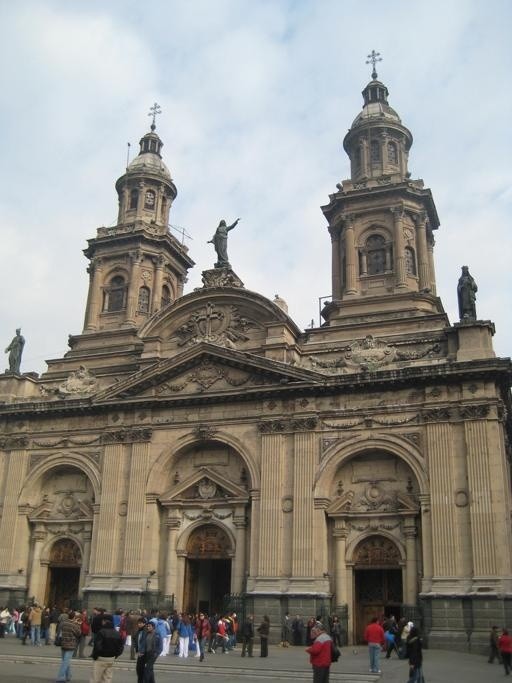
[488,625,502,665]
[208,217,241,264]
[455,263,478,317]
[5,329,26,372]
[495,627,512,676]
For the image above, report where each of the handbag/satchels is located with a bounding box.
[54,635,63,646]
[385,631,395,642]
[330,640,340,662]
